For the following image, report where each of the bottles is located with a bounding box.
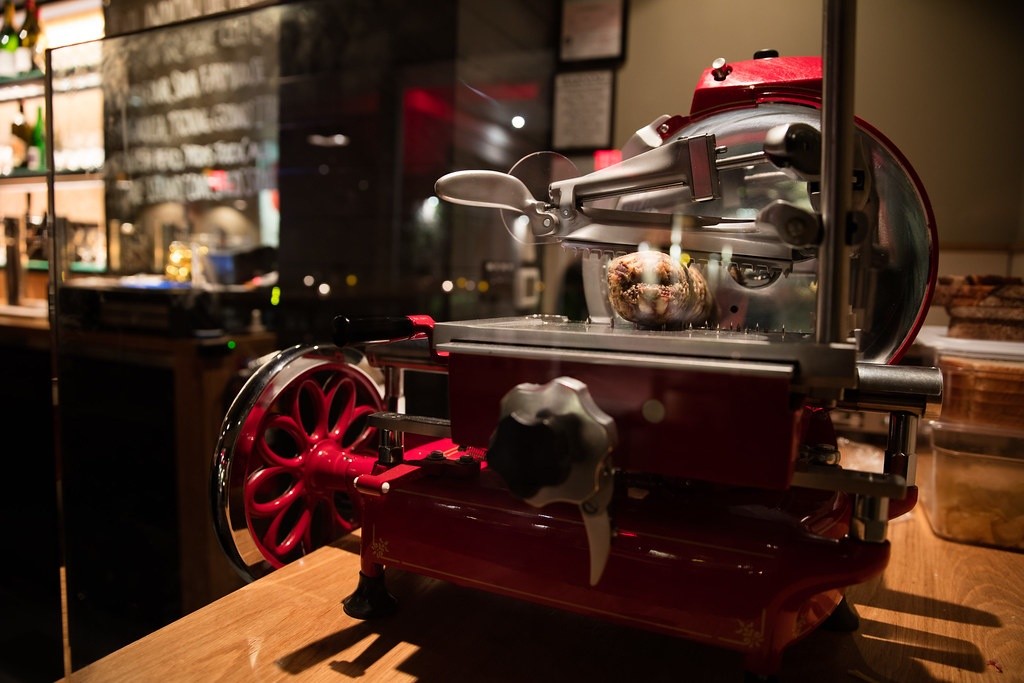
[24,192,31,227]
[11,105,30,168]
[0,0,19,78]
[18,0,41,70]
[28,108,46,173]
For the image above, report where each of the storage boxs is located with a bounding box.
[926,415,1024,550]
[934,355,1024,435]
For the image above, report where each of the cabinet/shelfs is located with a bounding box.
[0,0,111,319]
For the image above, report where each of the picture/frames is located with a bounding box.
[551,67,614,154]
[553,0,631,69]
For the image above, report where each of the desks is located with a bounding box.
[0,322,277,613]
